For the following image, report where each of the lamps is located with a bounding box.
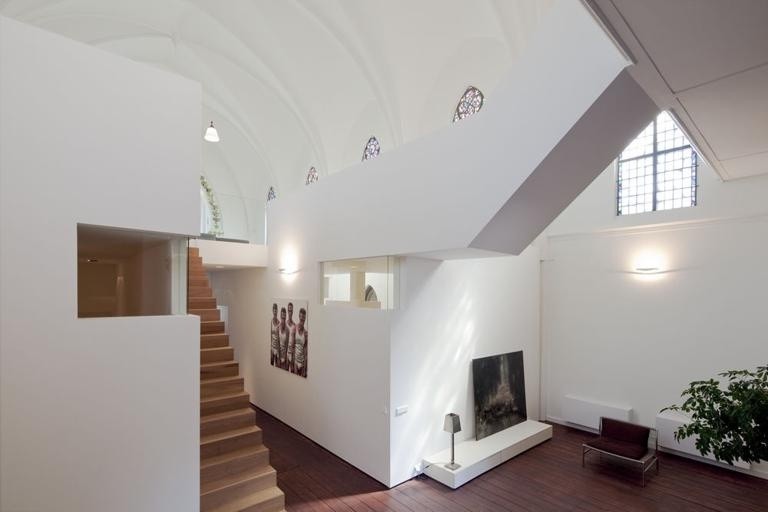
[204,122,219,141]
[443,414,461,470]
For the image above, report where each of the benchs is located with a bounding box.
[583,416,660,488]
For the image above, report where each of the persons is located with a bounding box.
[270,302,309,378]
